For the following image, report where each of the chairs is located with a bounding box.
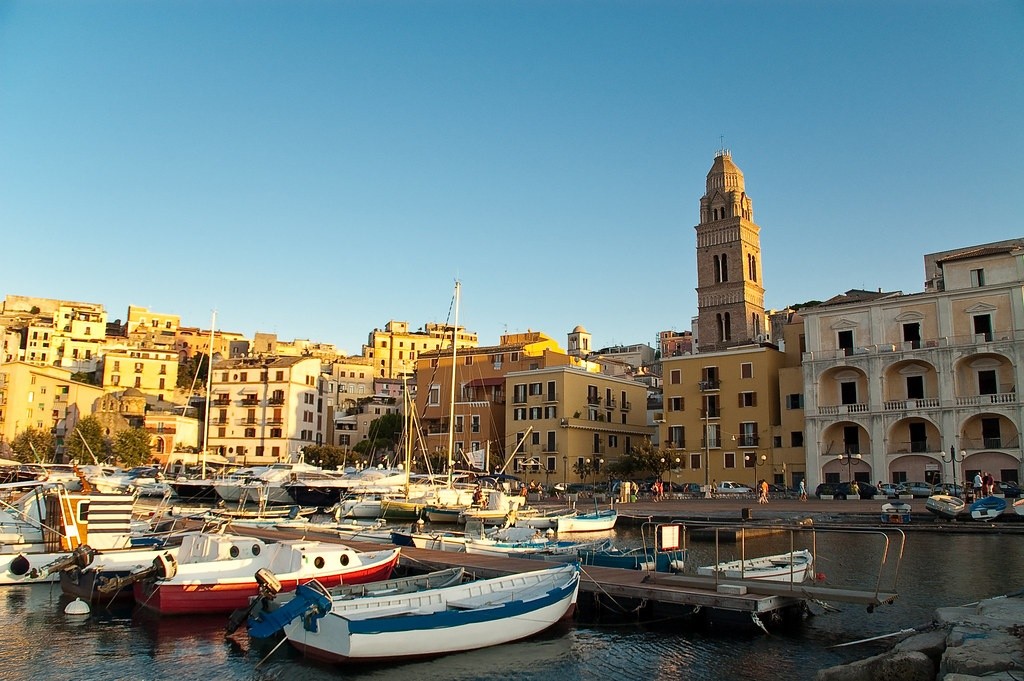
[918,483,920,487]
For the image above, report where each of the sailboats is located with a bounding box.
[378,280,526,520]
[162,309,220,502]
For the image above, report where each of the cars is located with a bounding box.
[815,481,877,500]
[554,479,807,501]
[934,480,1024,498]
[894,481,935,499]
[874,483,898,497]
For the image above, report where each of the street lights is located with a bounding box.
[586,456,604,503]
[941,445,966,497]
[563,454,568,495]
[745,450,767,500]
[660,454,682,495]
[837,446,862,495]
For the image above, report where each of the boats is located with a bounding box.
[393,531,814,587]
[1012,498,1024,516]
[925,493,965,519]
[249,563,585,669]
[969,495,1006,522]
[881,500,911,525]
[0,455,402,618]
[421,499,617,534]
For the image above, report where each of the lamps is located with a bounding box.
[731,435,736,440]
[597,391,602,402]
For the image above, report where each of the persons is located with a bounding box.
[684,484,689,493]
[974,472,983,499]
[529,479,542,493]
[982,472,989,497]
[877,481,882,490]
[988,474,994,496]
[757,479,768,504]
[799,478,807,500]
[520,482,528,505]
[713,479,718,490]
[849,481,861,495]
[630,479,665,502]
[473,486,483,505]
[941,485,950,496]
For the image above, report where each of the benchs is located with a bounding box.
[343,606,418,622]
[745,565,777,569]
